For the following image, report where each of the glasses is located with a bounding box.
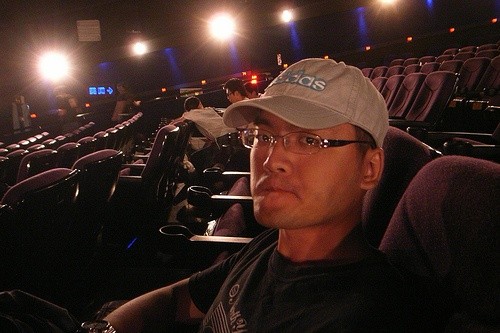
[240,128,375,156]
[225,92,235,98]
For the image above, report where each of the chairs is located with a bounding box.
[0,43,500,333]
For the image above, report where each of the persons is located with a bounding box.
[185,77,250,160]
[245,83,261,100]
[73,57,409,333]
[112,79,140,123]
[170,96,242,171]
[53,86,79,133]
[11,94,30,133]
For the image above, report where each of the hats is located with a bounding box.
[223,58,390,149]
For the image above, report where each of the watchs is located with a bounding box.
[75,319,117,333]
